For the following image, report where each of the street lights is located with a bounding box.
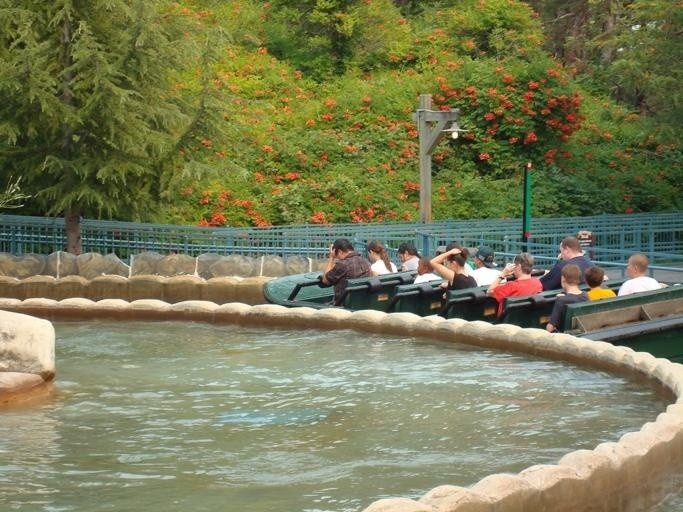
[411,94,470,224]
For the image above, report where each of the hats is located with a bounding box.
[476,245,497,269]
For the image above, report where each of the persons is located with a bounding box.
[395,243,420,271]
[583,267,617,302]
[413,257,438,285]
[537,236,607,291]
[364,239,397,274]
[430,247,477,300]
[320,238,373,306]
[486,251,543,318]
[443,243,473,276]
[470,245,506,288]
[546,264,590,334]
[617,254,660,296]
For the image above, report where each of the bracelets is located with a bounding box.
[499,275,504,281]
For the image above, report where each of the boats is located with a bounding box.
[263,269,683,365]
